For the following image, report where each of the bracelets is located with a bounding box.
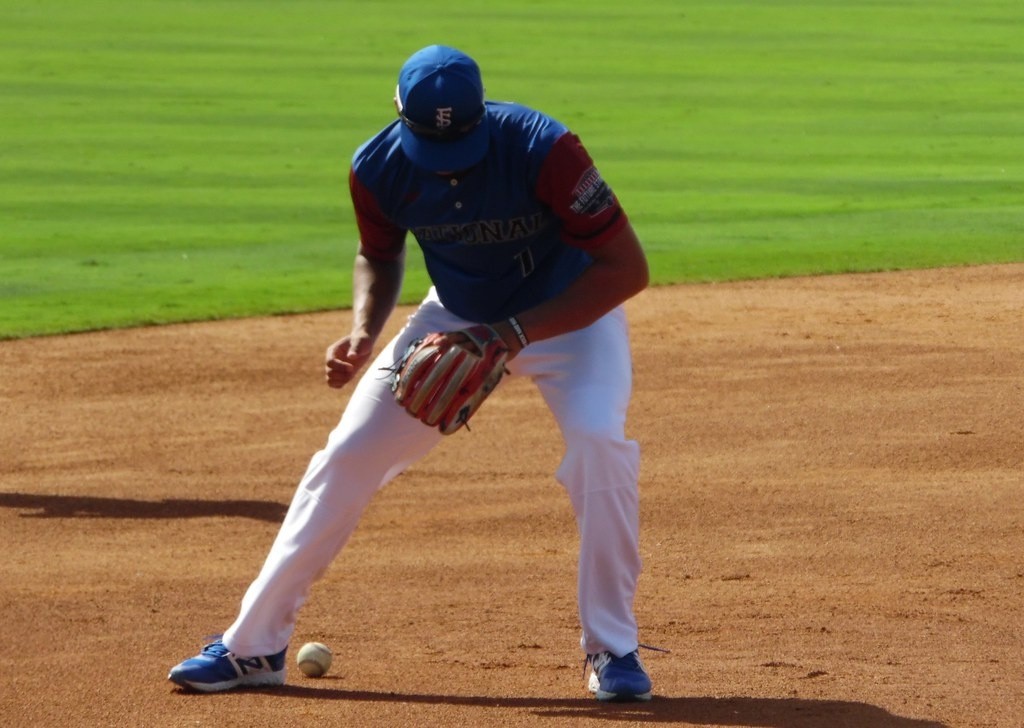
[507,316,529,349]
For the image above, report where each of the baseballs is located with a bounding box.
[298,642,333,678]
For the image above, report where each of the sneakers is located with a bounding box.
[167,641,288,691]
[589,649,652,700]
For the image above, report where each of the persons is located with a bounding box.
[166,43,652,702]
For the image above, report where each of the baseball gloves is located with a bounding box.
[391,324,511,436]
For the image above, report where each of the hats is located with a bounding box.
[396,45,490,171]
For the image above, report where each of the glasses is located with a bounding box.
[395,104,485,141]
